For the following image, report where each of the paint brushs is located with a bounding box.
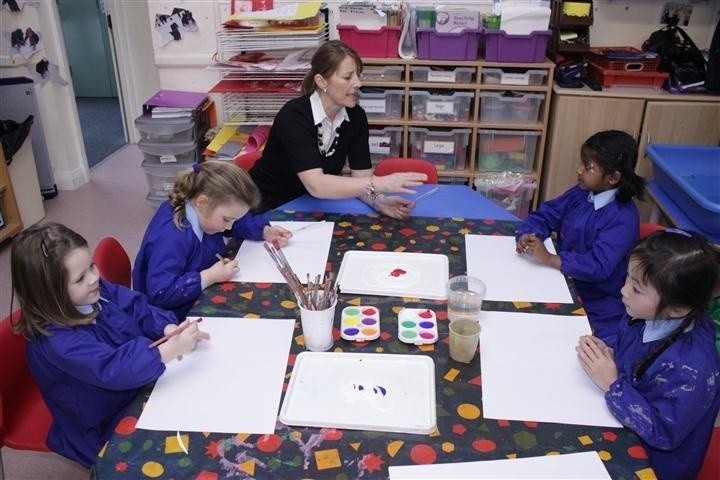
[148,318,203,348]
[264,239,340,311]
[291,220,326,234]
[216,253,226,264]
[404,185,442,209]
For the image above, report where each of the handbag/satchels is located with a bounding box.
[641,25,706,84]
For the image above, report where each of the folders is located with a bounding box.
[143,90,209,121]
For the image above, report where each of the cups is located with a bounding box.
[447,276,488,321]
[296,290,339,353]
[448,320,482,363]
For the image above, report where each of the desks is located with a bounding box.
[642,179,720,251]
[92,184,659,480]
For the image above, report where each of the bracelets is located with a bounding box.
[370,192,383,208]
[366,175,374,198]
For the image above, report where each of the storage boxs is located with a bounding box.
[134,115,197,211]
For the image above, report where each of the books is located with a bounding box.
[681,80,705,90]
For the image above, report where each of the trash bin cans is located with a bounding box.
[0,114,47,234]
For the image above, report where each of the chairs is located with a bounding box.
[696,427,720,480]
[640,222,663,241]
[0,309,55,479]
[374,158,438,184]
[90,236,132,289]
[235,151,261,172]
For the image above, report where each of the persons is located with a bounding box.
[514,129,647,346]
[575,227,720,480]
[248,39,415,221]
[10,221,211,476]
[132,161,294,322]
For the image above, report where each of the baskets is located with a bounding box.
[590,45,659,72]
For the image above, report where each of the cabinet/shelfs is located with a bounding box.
[212,8,332,153]
[0,141,24,244]
[542,94,720,204]
